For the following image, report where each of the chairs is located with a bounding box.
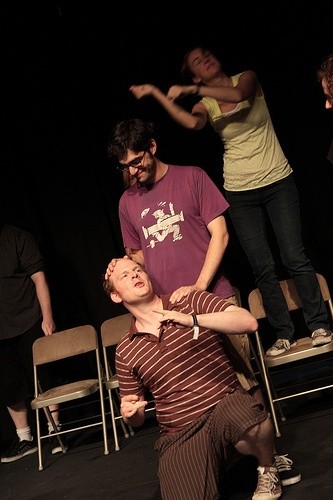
[101,313,155,451]
[248,273,333,437]
[31,325,130,471]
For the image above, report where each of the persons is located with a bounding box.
[104,117,302,486]
[0,225,67,463]
[102,259,283,500]
[319,56,333,109]
[129,46,333,357]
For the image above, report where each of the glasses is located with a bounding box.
[116,144,150,174]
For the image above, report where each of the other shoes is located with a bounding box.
[0,436,38,464]
[49,423,69,454]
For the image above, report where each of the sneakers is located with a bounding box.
[251,460,281,500]
[311,329,333,347]
[273,451,301,486]
[265,337,297,358]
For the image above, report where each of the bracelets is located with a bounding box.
[190,313,199,340]
